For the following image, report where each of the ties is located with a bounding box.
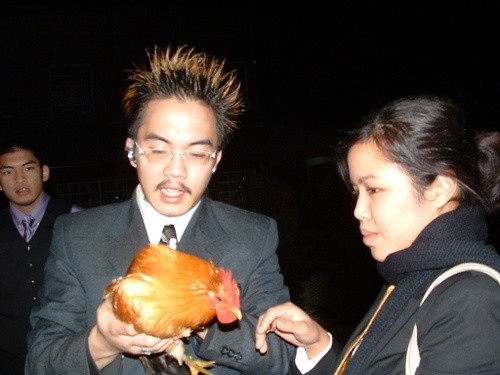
[21,215,35,242]
[158,225,179,252]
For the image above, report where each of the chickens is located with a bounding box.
[99,242,245,375]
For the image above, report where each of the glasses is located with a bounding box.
[134,141,217,166]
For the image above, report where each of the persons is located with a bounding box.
[0,144,87,375]
[254,93,500,375]
[23,44,295,375]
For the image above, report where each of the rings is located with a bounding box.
[142,348,151,355]
